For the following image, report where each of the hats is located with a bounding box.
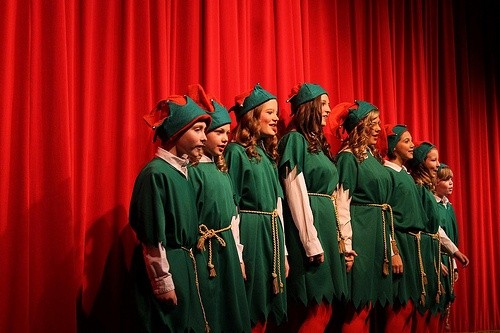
[143,93,211,140]
[328,99,379,143]
[385,123,408,156]
[286,81,329,107]
[437,163,448,173]
[234,81,277,117]
[188,84,232,134]
[409,140,435,171]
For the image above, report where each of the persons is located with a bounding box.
[228,83,289,333]
[381,125,469,333]
[190,95,250,333]
[337,99,389,333]
[129,95,212,333]
[276,81,357,333]
